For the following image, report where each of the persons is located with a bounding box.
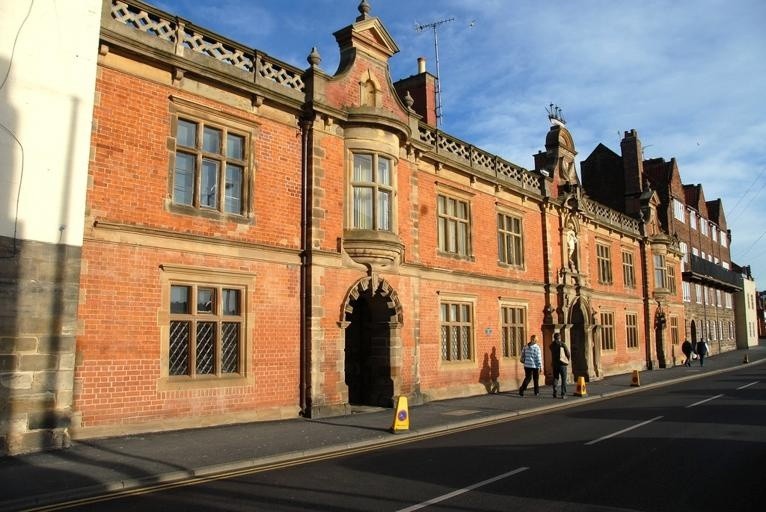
[567,223,576,265]
[697,338,709,366]
[549,334,569,399]
[519,335,543,396]
[682,337,694,367]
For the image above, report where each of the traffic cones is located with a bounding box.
[629,369,641,387]
[389,395,411,435]
[573,376,589,398]
[742,353,750,363]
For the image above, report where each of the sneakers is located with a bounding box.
[563,396,568,400]
[536,393,541,396]
[519,387,523,397]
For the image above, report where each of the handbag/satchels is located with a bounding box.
[520,352,525,363]
[559,347,569,366]
